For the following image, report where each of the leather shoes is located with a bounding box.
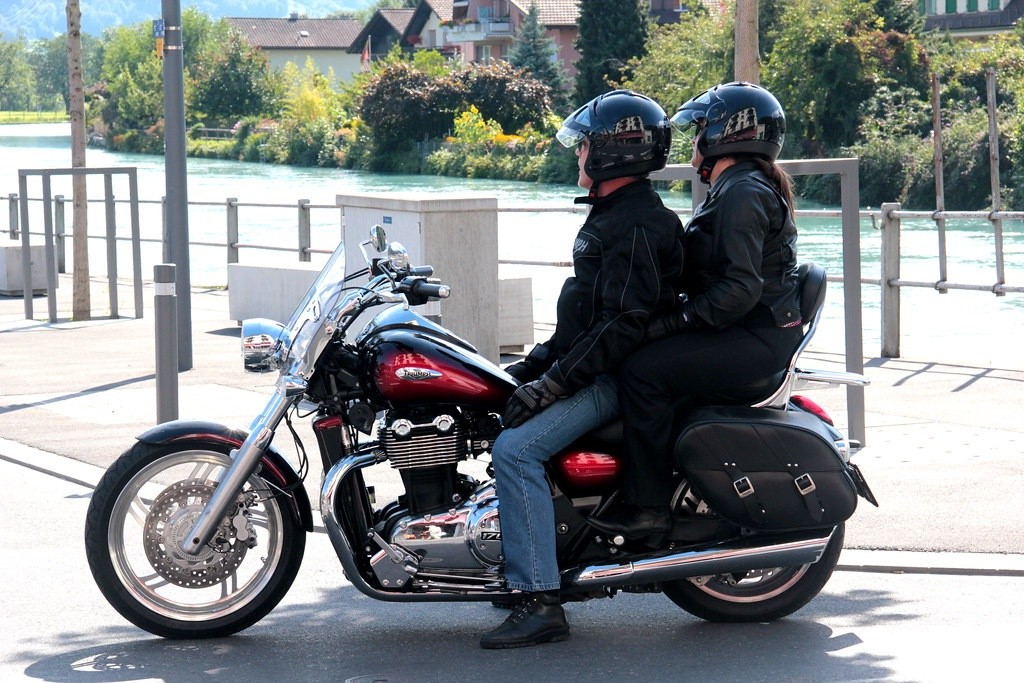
[480,594,570,651]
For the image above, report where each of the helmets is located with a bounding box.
[562,90,672,182]
[678,82,786,162]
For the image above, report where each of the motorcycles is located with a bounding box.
[84,224,879,640]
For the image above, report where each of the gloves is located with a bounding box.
[501,361,569,429]
[648,312,689,343]
[503,343,557,384]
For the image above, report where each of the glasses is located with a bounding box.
[578,141,590,152]
[695,117,706,137]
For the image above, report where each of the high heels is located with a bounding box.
[585,507,672,550]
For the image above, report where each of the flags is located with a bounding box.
[360,40,369,61]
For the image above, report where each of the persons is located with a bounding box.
[477,89,684,649]
[584,81,805,546]
